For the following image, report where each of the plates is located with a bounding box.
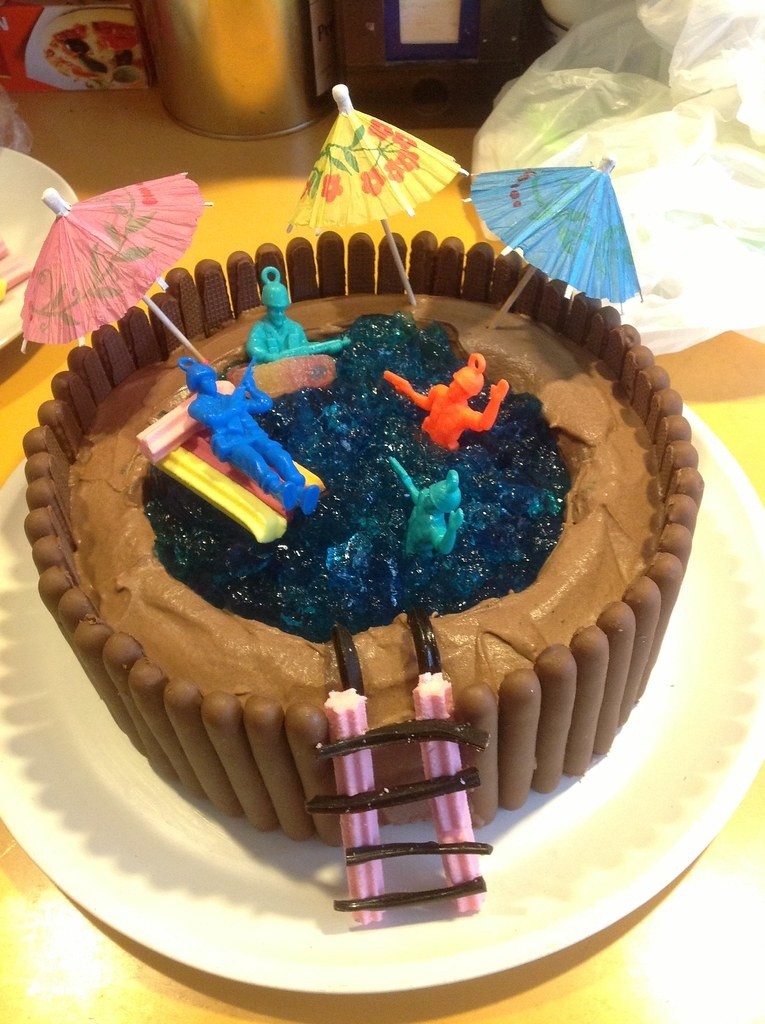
[0,147,82,350]
[0,370,765,995]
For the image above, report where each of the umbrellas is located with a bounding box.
[286,83,461,306]
[20,171,206,364]
[468,156,645,335]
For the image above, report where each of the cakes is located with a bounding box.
[23,226,707,927]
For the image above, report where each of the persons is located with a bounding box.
[247,268,352,364]
[383,351,509,451]
[388,455,463,558]
[178,356,319,514]
